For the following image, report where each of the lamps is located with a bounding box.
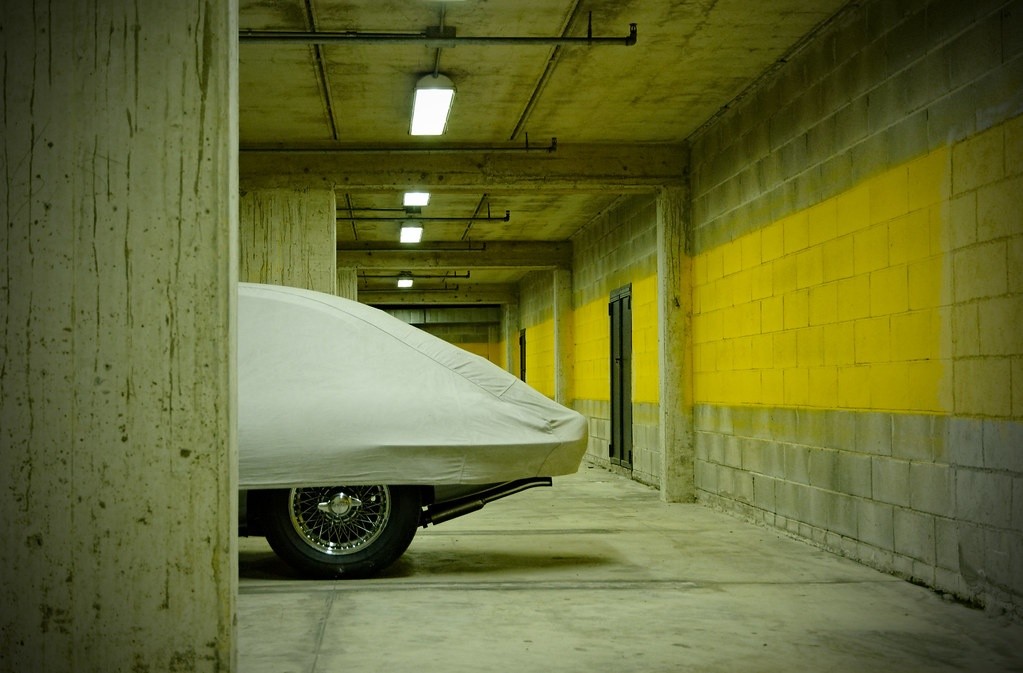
[398,271,413,288]
[403,193,430,206]
[400,222,423,243]
[408,74,456,136]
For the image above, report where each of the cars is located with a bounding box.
[237,282,588,577]
